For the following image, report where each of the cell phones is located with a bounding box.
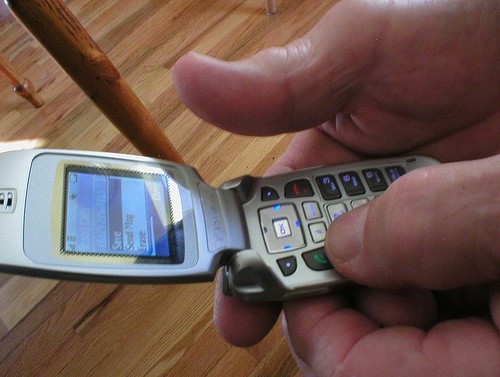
[1,149,447,301]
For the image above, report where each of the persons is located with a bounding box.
[172,0,500,377]
